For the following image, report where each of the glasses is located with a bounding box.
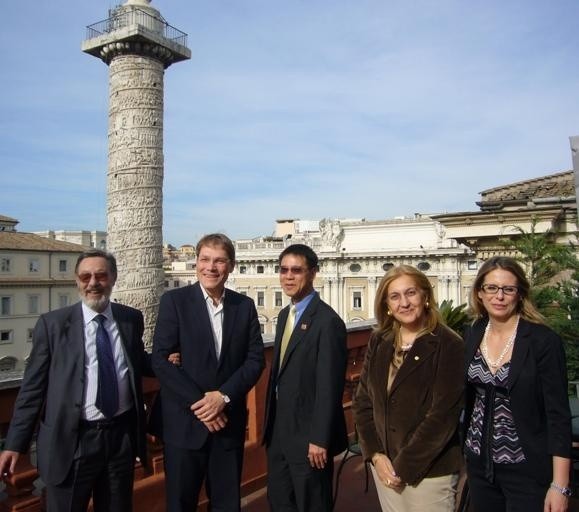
[476,283,521,297]
[279,266,309,275]
[75,271,109,283]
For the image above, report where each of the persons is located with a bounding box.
[1,246,183,511]
[265,243,354,512]
[149,232,266,512]
[463,257,575,511]
[352,262,469,512]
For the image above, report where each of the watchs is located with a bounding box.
[219,390,231,405]
[550,481,573,498]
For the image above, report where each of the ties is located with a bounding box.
[94,314,119,420]
[276,305,297,367]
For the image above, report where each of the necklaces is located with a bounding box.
[484,322,515,367]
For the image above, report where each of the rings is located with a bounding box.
[385,479,391,486]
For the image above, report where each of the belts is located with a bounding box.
[81,417,127,428]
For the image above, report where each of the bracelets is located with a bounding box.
[368,455,383,467]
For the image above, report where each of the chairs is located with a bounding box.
[332,377,371,504]
